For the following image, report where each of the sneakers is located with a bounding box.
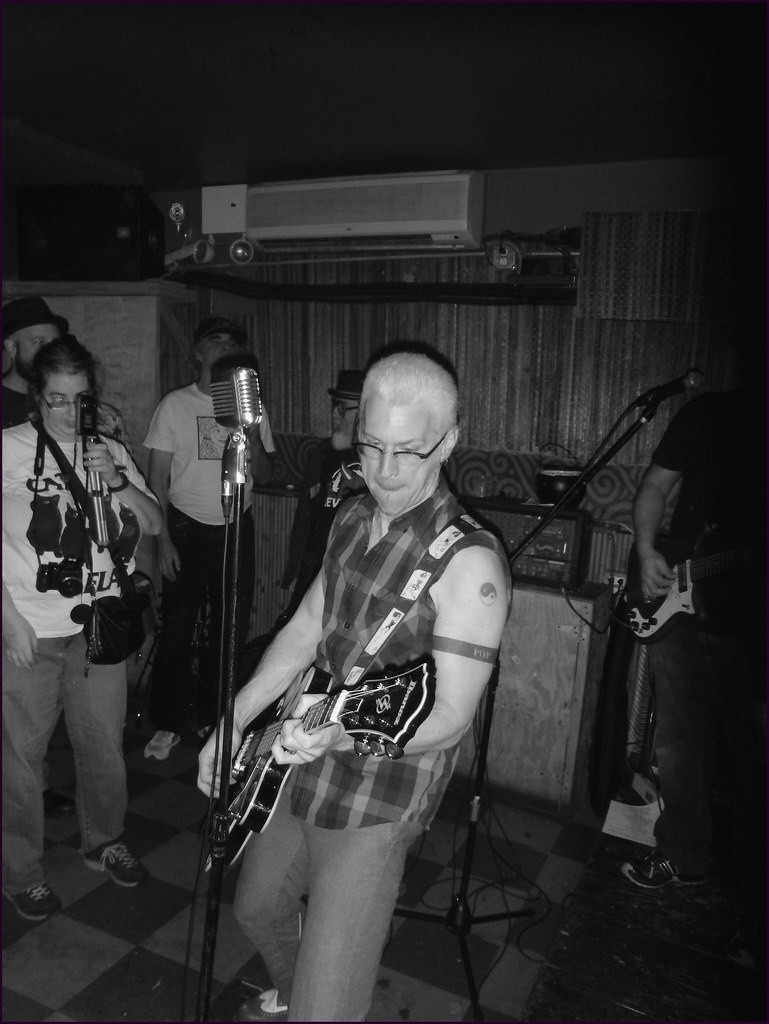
[142,730,186,763]
[2,881,62,921]
[726,895,764,971]
[621,850,707,887]
[84,837,149,889]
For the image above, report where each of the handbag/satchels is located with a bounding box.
[88,592,146,665]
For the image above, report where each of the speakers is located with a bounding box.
[445,579,616,824]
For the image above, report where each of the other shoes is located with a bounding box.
[233,985,291,1023]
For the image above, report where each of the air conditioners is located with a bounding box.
[246,171,486,252]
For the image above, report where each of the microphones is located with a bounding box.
[637,371,705,408]
[210,366,263,516]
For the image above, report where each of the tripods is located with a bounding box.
[391,393,673,1024]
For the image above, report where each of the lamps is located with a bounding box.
[194,186,253,264]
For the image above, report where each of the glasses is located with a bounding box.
[331,402,360,417]
[352,420,446,467]
[37,391,80,412]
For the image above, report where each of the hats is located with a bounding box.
[326,368,366,401]
[1,294,70,348]
[194,317,244,343]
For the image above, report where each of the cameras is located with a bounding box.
[36,557,83,598]
[75,395,98,436]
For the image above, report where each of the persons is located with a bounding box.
[196,355,513,1023]
[144,316,276,741]
[1,294,75,818]
[1,336,161,918]
[282,369,368,618]
[620,387,768,890]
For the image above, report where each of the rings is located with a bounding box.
[290,750,297,754]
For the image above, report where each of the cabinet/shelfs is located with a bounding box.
[455,582,613,818]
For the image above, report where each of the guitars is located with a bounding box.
[625,536,769,647]
[207,651,438,871]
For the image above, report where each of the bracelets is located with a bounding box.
[108,472,128,494]
